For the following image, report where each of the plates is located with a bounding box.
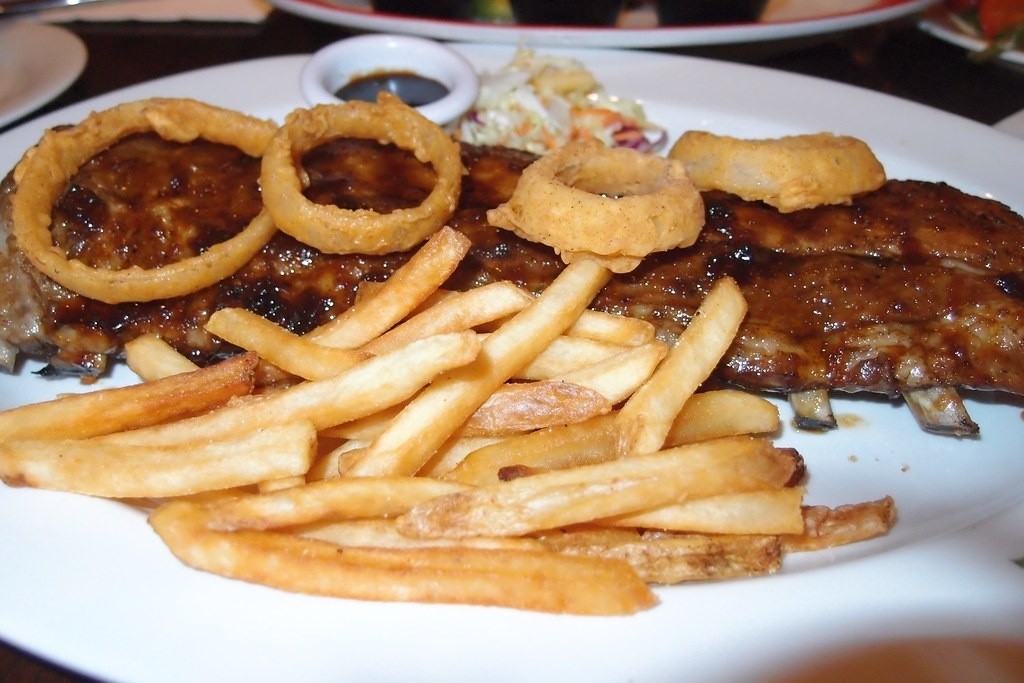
[0,47,1024,683]
[0,23,87,129]
[270,0,944,44]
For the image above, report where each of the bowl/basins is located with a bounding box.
[303,32,478,134]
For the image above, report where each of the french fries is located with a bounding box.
[0,229,894,620]
